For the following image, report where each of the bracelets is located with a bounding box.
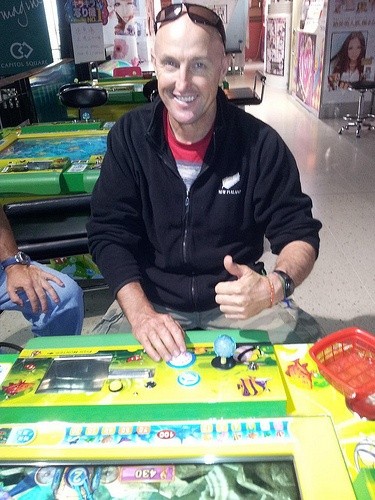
[267,277,274,308]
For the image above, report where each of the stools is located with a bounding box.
[339,80,375,138]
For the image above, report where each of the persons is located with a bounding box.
[113,0,142,34]
[0,195,83,337]
[332,32,366,82]
[85,4,322,363]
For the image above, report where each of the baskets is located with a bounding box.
[309,327,375,399]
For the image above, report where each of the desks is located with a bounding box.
[0,121,115,214]
[0,344,375,500]
[67,76,151,118]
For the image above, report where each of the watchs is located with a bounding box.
[1,252,30,270]
[274,270,295,298]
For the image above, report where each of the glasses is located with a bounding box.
[154,3,227,54]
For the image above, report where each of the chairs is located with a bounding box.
[225,69,266,112]
[59,86,108,120]
[225,40,244,74]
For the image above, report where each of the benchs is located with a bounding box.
[5,212,89,253]
